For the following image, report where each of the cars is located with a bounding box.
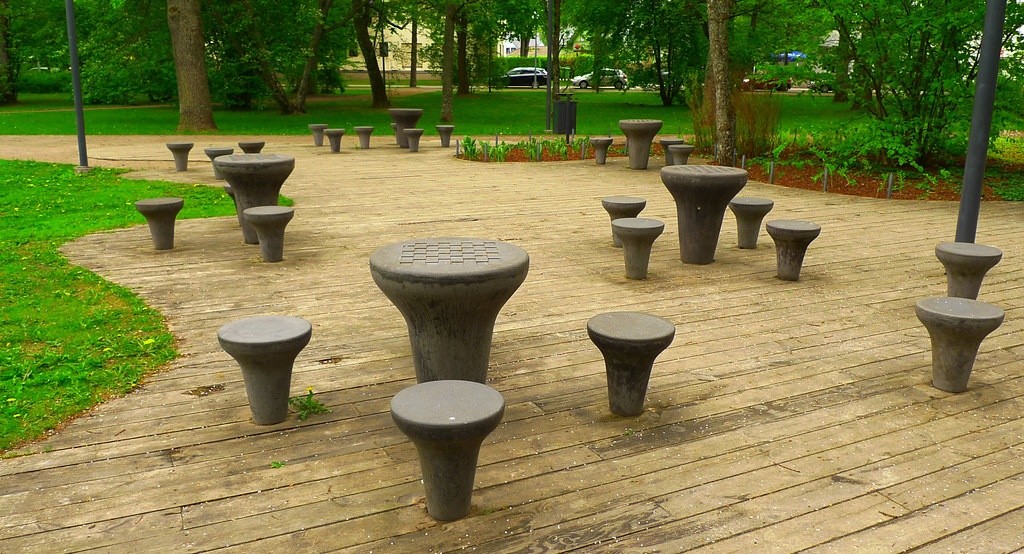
[808,76,834,92]
[573,68,628,89]
[742,73,793,92]
[498,66,547,86]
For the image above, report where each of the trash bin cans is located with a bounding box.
[552,94,577,134]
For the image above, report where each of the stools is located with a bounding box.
[309,124,373,154]
[590,137,695,169]
[134,197,185,250]
[766,219,820,282]
[388,380,505,520]
[728,197,773,250]
[917,296,1005,392]
[602,197,646,246]
[168,142,266,179]
[610,218,663,279]
[217,316,311,427]
[586,310,675,416]
[390,123,455,151]
[223,181,293,262]
[935,242,1003,301]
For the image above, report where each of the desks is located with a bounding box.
[619,120,662,170]
[662,164,747,263]
[216,154,295,246]
[367,238,529,384]
[389,107,423,148]
